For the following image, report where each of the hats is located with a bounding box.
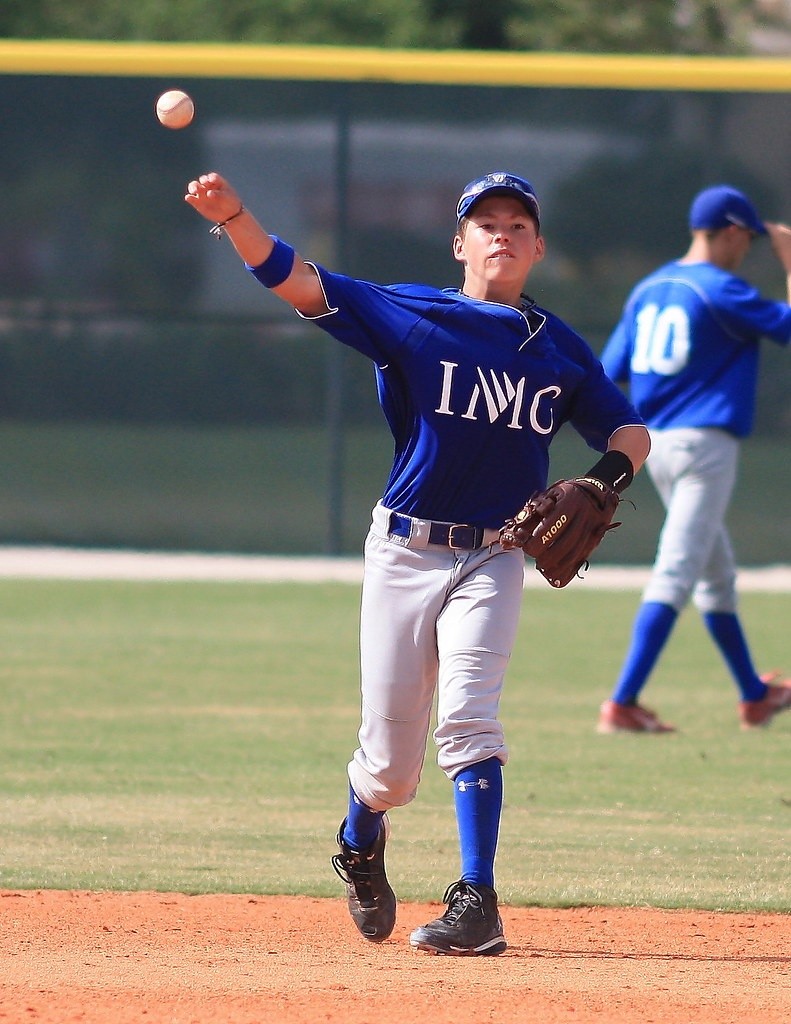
[689,184,768,237]
[457,172,541,228]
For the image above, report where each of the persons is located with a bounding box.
[595,186,791,737]
[183,170,653,958]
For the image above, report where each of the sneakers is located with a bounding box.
[599,700,676,735]
[737,680,791,728]
[331,813,396,943]
[410,879,507,956]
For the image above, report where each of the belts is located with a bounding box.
[388,512,503,550]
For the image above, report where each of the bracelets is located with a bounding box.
[209,204,245,240]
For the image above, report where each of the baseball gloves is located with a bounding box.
[486,474,637,591]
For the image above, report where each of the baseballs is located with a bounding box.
[154,89,196,130]
